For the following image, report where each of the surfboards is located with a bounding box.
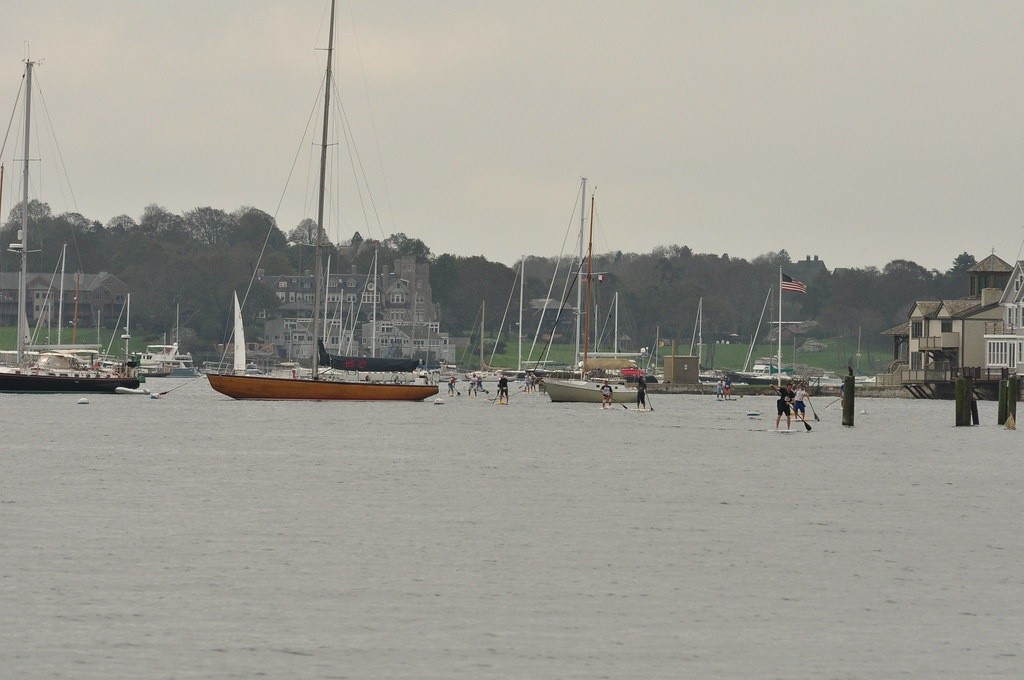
[767,419,814,432]
[716,398,737,401]
[598,407,651,412]
[497,400,512,405]
[446,394,478,399]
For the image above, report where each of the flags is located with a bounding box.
[782,272,808,294]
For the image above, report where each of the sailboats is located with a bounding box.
[541,179,643,406]
[0,55,145,396]
[20,246,840,379]
[207,0,440,403]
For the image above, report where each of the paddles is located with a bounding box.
[492,388,501,404]
[771,386,820,430]
[609,389,654,411]
[452,385,461,395]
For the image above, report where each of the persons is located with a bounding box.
[635,379,647,410]
[724,381,731,400]
[499,374,509,404]
[476,375,484,391]
[464,372,478,397]
[775,383,796,433]
[716,377,724,400]
[524,371,537,393]
[497,377,504,397]
[365,375,369,381]
[447,375,456,396]
[839,382,846,410]
[792,382,810,422]
[599,380,612,408]
[394,376,402,384]
